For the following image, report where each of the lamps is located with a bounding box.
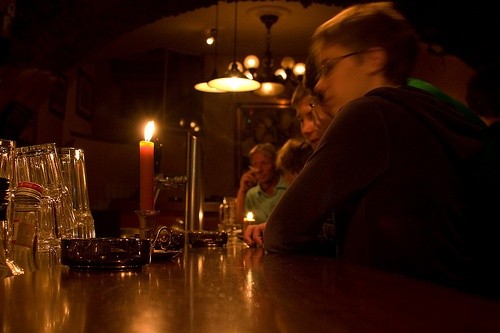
[194,1,306,97]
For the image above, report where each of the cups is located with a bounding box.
[0,139,95,279]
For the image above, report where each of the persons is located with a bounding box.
[235,2,499,299]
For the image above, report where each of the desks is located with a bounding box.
[1,236,500,332]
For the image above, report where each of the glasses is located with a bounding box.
[311,50,366,82]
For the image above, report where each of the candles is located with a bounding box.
[140,120,154,211]
